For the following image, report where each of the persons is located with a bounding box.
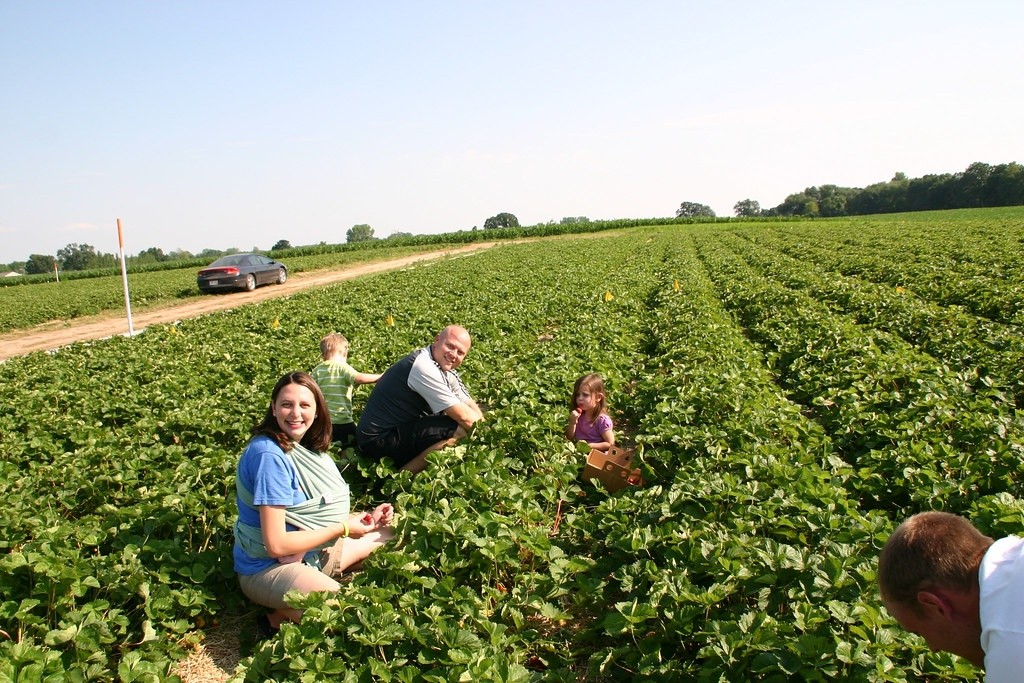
[233,372,401,657]
[278,528,308,565]
[354,324,485,482]
[310,334,385,449]
[877,511,1024,683]
[565,373,616,454]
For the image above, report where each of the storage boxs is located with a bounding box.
[579,444,643,496]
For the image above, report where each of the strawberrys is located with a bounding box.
[576,407,582,413]
[364,513,372,521]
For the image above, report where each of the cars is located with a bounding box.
[197,254,288,292]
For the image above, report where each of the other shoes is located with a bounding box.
[258,612,281,640]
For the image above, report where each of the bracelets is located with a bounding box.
[340,521,349,538]
